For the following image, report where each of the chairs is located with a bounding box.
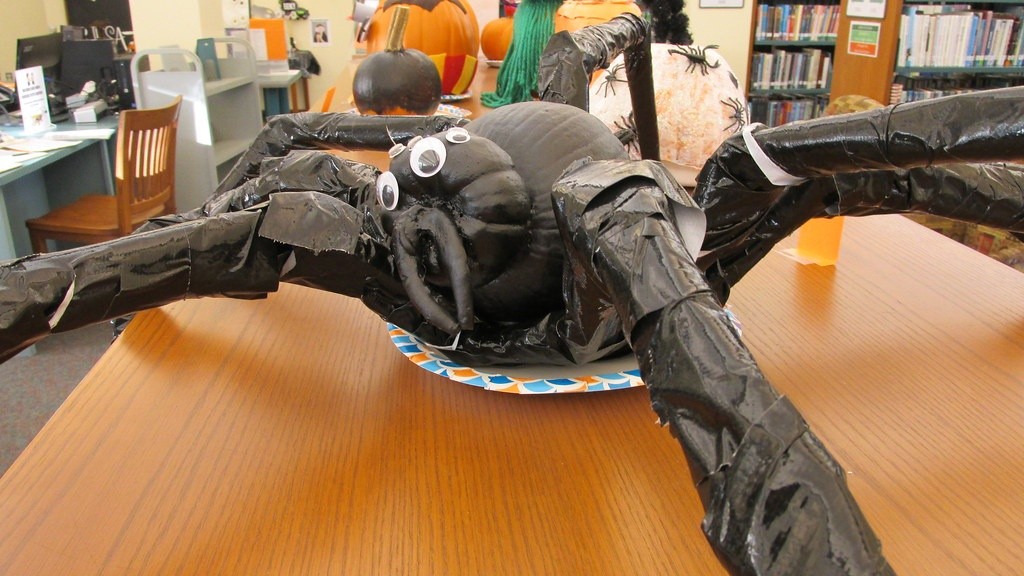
[25,94,184,253]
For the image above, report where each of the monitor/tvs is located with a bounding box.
[15,33,117,105]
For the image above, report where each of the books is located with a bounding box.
[889,5,1024,105]
[750,4,840,128]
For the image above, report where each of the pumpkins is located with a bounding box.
[482,17,515,62]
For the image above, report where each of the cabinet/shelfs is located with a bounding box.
[745,1,840,129]
[130,36,262,212]
[831,0,1024,107]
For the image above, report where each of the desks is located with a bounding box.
[0,61,1024,576]
[0,113,119,266]
[257,69,302,115]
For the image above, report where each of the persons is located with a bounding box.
[314,24,327,42]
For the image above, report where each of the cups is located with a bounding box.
[796,215,844,267]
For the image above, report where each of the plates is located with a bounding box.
[481,58,503,67]
[440,90,472,103]
[388,307,741,396]
[343,104,472,120]
